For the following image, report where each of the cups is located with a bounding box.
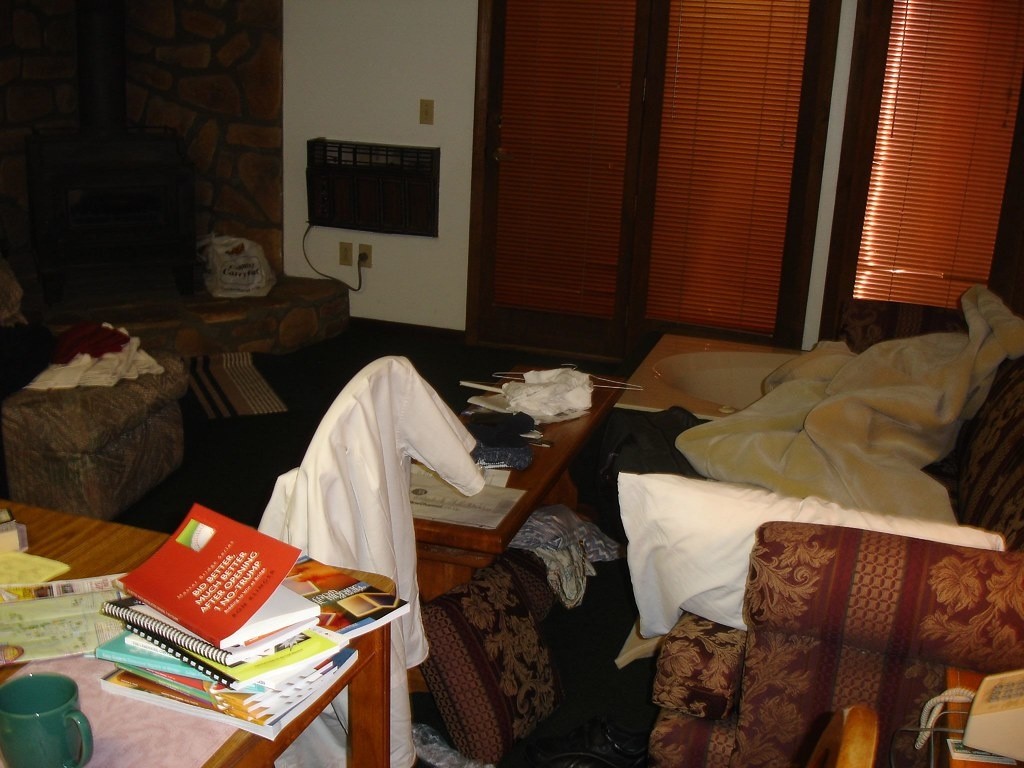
[0,672,94,768]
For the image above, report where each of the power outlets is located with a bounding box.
[419,99,434,125]
[339,242,353,266]
[359,243,373,268]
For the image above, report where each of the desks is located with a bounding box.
[0,499,396,768]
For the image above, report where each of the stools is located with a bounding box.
[1,352,190,523]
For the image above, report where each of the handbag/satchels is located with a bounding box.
[203,237,274,296]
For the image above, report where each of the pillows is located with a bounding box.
[419,549,584,764]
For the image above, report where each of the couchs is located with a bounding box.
[648,295,1023,768]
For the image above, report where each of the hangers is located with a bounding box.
[491,363,645,393]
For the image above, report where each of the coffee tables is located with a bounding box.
[408,363,630,604]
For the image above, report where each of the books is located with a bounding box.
[1,502,412,741]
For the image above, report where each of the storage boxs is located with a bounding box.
[26,123,206,306]
